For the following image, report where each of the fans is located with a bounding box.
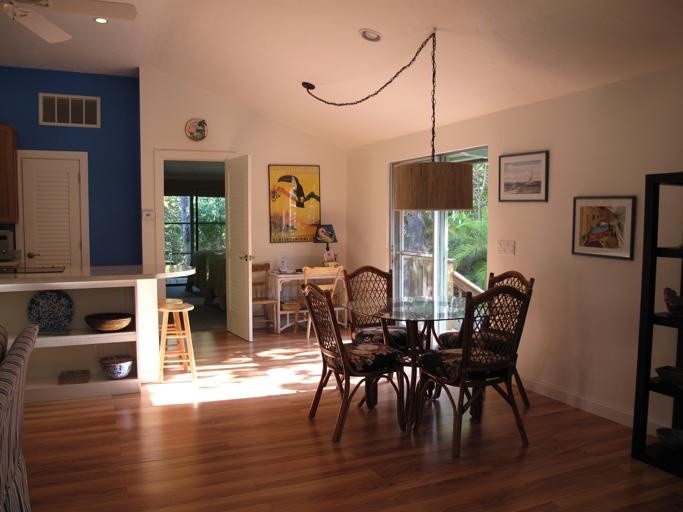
[2,2,138,43]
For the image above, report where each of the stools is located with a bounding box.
[157,296,188,374]
[155,301,199,382]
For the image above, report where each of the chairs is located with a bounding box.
[408,286,531,459]
[338,263,430,416]
[294,266,342,340]
[293,281,406,444]
[431,268,535,419]
[250,263,278,336]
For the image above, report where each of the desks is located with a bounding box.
[334,287,519,428]
[266,269,349,335]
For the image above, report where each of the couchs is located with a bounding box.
[0,321,49,512]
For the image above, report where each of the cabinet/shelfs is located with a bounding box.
[630,169,682,480]
[1,121,21,225]
[0,267,196,406]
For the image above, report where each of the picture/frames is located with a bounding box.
[266,162,321,244]
[570,193,637,262]
[497,151,549,205]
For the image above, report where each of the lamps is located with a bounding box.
[312,223,337,262]
[301,28,473,212]
